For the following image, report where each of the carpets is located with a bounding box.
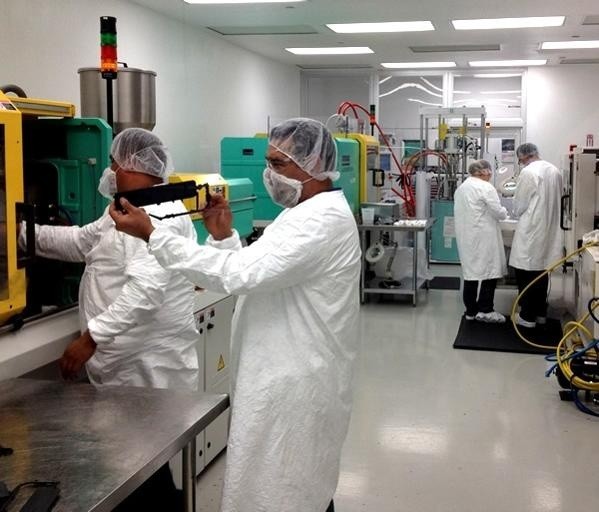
[453,313,567,355]
[420,276,461,290]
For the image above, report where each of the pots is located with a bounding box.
[78,62,157,133]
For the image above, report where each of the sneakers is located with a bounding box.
[537,317,546,325]
[466,316,475,320]
[475,310,506,323]
[511,313,536,328]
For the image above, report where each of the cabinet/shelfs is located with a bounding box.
[358,218,438,309]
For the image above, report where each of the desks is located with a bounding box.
[0,376,232,512]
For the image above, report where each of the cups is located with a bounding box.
[361,207,374,224]
[586,134,593,147]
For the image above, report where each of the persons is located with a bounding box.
[508,144,563,327]
[108,117,362,512]
[454,160,509,323]
[1,126,199,511]
